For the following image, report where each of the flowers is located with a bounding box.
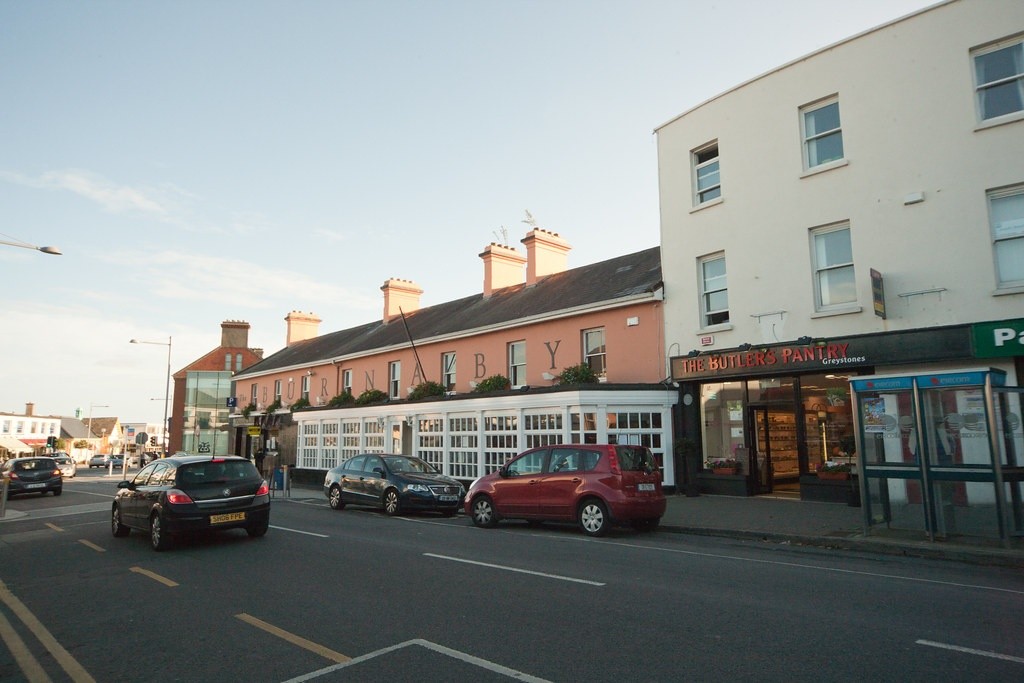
[815,462,851,473]
[704,458,738,468]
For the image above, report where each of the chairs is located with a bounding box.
[204,466,223,479]
[555,456,570,471]
[34,462,46,469]
[184,471,196,480]
[16,463,24,470]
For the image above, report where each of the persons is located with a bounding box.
[152,451,158,461]
[254,448,265,475]
[140,451,146,468]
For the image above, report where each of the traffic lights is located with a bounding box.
[150,436,155,446]
[46,436,51,447]
[52,437,58,447]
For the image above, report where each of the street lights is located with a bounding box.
[151,394,172,417]
[85,406,109,466]
[130,336,172,459]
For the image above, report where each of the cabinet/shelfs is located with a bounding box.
[759,422,849,476]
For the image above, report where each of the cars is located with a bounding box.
[111,454,271,551]
[89,454,110,468]
[105,454,124,468]
[464,444,667,538]
[53,457,76,478]
[0,457,62,501]
[324,453,466,517]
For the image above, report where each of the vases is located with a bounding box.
[817,471,848,481]
[713,468,736,475]
